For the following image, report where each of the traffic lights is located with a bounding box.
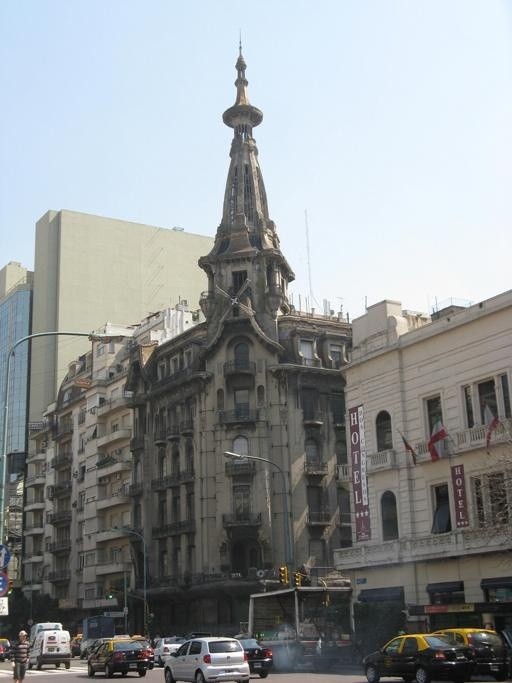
[294,574,301,587]
[279,567,288,583]
[106,585,113,600]
[7,581,13,596]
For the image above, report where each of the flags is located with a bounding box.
[400,433,418,466]
[483,404,499,456]
[427,416,450,463]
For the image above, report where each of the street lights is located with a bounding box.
[110,527,148,635]
[0,332,126,545]
[222,451,294,571]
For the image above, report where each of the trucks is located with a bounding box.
[234,587,355,671]
[77,616,114,659]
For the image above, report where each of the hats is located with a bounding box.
[18,629,29,636]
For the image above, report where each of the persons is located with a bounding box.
[10,630,30,683]
[484,622,493,630]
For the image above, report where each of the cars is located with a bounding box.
[362,627,512,683]
[26,622,273,683]
[0,638,12,661]
